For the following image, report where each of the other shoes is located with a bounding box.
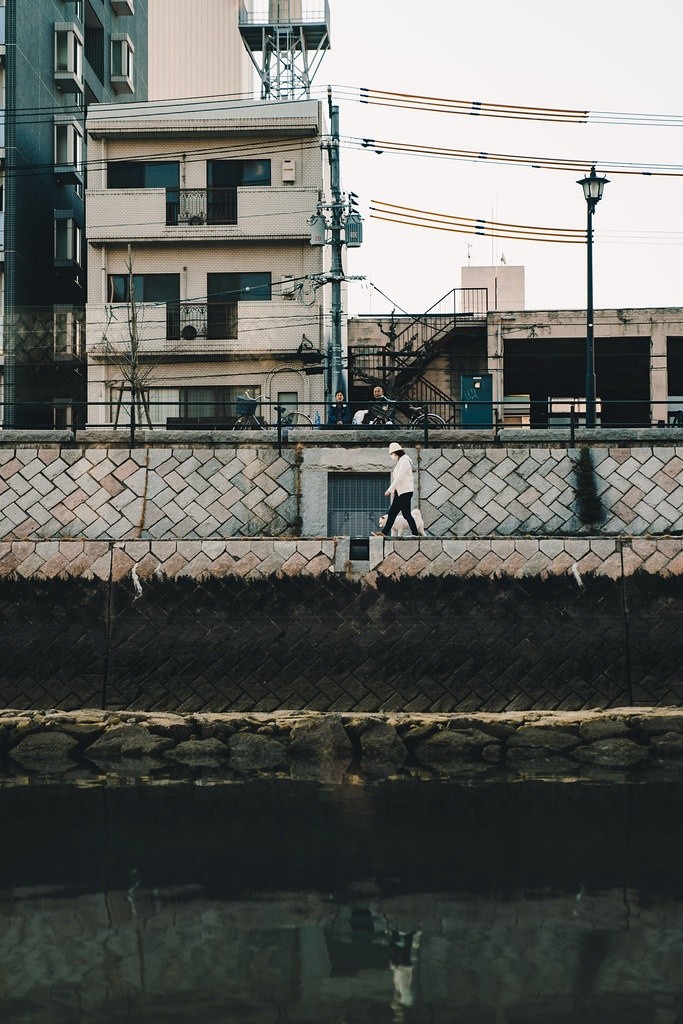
[371,531,385,536]
[405,534,419,537]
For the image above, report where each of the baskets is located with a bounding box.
[236,396,257,415]
[369,402,396,419]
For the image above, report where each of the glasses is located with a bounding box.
[390,453,395,455]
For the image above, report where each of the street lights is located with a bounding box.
[576,165,612,429]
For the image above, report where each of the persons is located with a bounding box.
[362,387,394,422]
[326,391,351,424]
[371,441,423,537]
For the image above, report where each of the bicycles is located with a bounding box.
[371,394,448,430]
[231,388,315,431]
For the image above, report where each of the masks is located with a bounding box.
[391,453,398,462]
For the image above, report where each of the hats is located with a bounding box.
[388,443,404,454]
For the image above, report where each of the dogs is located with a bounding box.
[378,508,427,537]
